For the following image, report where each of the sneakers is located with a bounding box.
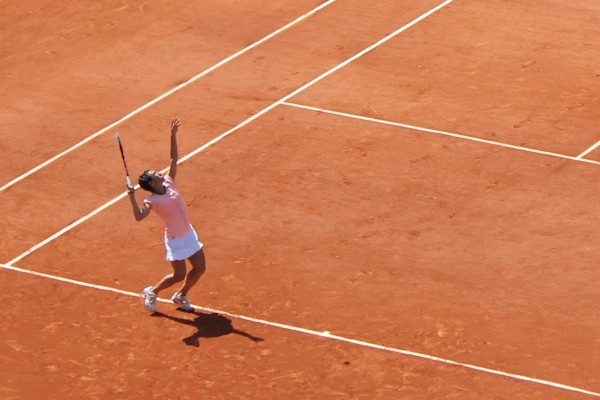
[171,293,193,311]
[143,286,159,313]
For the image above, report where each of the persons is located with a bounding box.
[125,117,206,313]
